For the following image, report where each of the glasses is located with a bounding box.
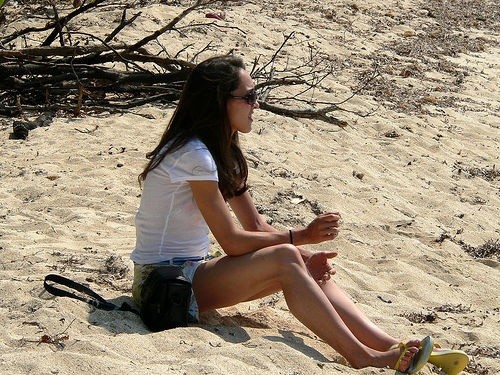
[231,90,258,105]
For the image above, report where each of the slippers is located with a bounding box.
[387,335,469,374]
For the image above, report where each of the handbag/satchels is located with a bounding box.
[139,265,191,332]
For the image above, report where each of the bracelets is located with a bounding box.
[289,230,293,244]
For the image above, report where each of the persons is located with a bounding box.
[128,54,470,375]
